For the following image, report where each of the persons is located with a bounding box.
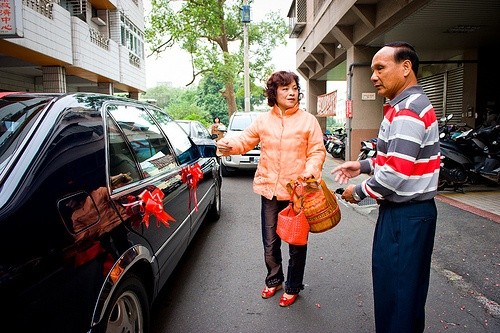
[211,115,227,142]
[217,70,326,306]
[330,41,441,332]
[64,172,140,267]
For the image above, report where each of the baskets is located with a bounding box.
[287,178,341,233]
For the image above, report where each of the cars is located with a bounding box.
[173,120,218,158]
[0,92,222,333]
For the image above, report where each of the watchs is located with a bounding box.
[351,185,361,202]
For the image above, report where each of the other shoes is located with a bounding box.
[279,292,298,307]
[262,284,282,299]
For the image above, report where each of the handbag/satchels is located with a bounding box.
[276,181,310,245]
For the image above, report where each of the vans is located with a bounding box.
[218,111,268,177]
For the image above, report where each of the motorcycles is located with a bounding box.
[356,112,500,193]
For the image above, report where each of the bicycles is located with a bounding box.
[323,128,348,160]
